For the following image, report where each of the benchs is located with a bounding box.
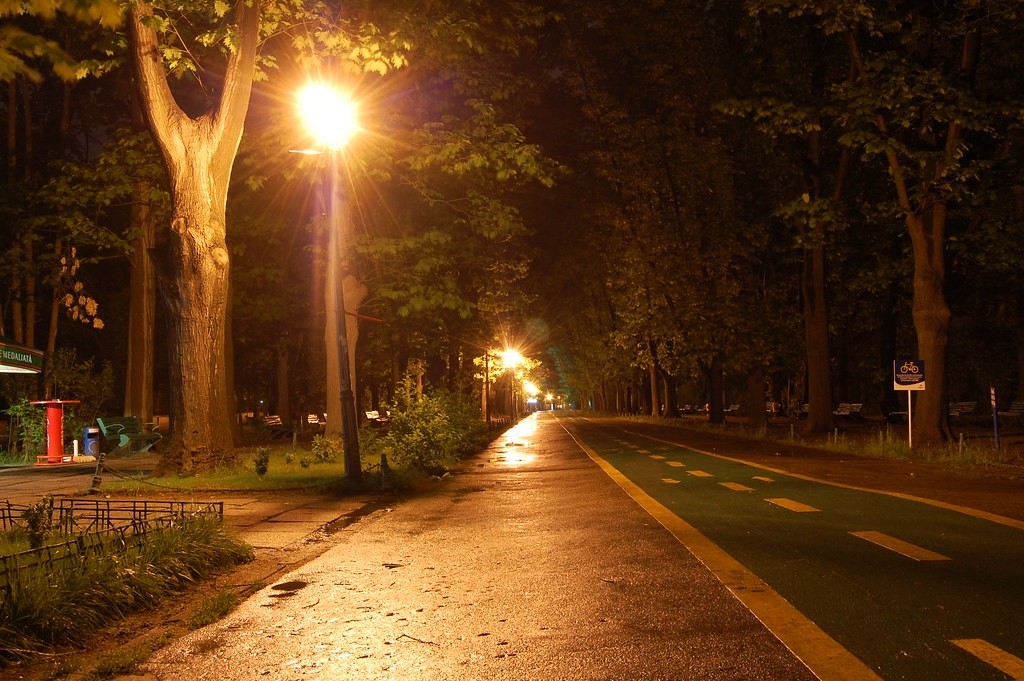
[945,401,980,421]
[784,403,809,417]
[724,405,740,418]
[890,408,917,425]
[96,416,162,459]
[661,403,707,415]
[364,410,390,425]
[834,403,862,423]
[304,413,328,425]
[991,400,1024,426]
[765,402,781,416]
[264,413,283,427]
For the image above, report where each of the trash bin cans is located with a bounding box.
[85,427,100,459]
